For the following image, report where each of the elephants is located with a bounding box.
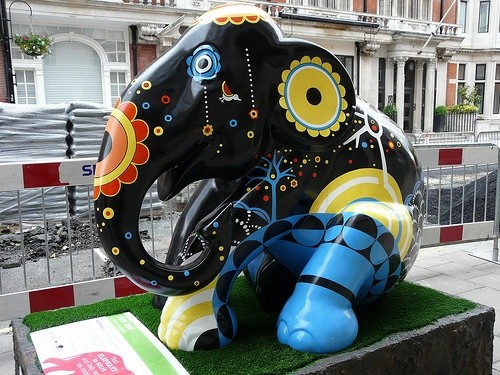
[93,2,425,354]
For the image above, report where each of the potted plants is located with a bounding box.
[14,33,55,59]
[435,105,446,132]
[382,105,396,123]
